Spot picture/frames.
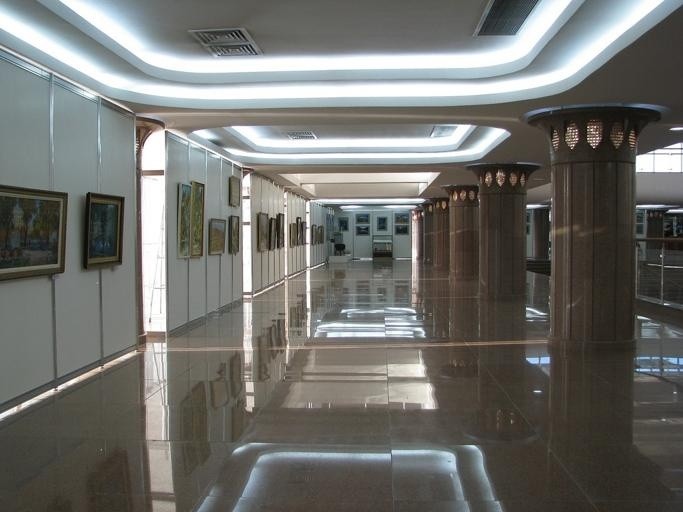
[177,176,410,259]
[84,191,125,270]
[46,269,410,512]
[0,184,68,281]
